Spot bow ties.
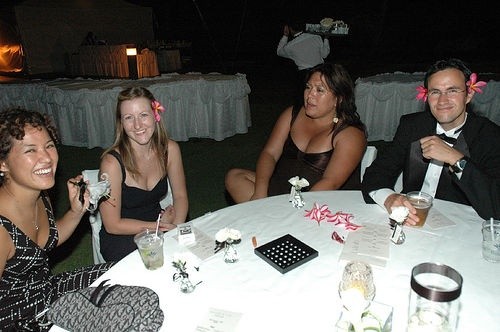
[435,133,457,145]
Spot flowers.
[215,227,241,244]
[304,202,366,230]
[386,205,409,224]
[416,85,429,103]
[75,173,115,211]
[465,73,487,94]
[151,100,166,121]
[288,176,310,190]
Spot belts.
[301,64,322,72]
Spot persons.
[277,15,330,93]
[225,63,367,204]
[361,56,500,230]
[0,108,99,332]
[98,86,189,262]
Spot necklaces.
[1,190,41,231]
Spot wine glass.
[339,262,376,332]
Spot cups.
[404,191,433,228]
[133,229,164,271]
[408,262,463,332]
[481,220,500,264]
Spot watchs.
[451,155,470,173]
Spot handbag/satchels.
[47,279,164,332]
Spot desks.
[354,71,500,142]
[48,190,500,332]
[0,72,252,150]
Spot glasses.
[425,89,466,98]
